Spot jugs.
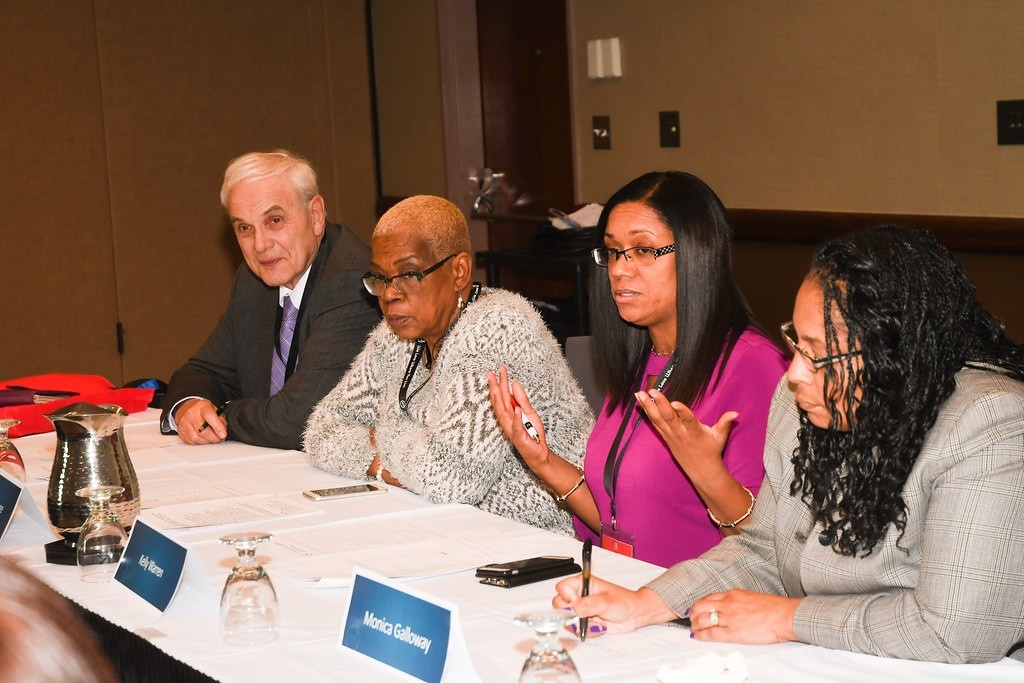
[43,401,142,549]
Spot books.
[0,389,80,407]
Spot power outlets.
[658,110,681,148]
[996,99,1024,146]
[591,114,612,151]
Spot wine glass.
[512,608,580,683]
[75,484,127,583]
[0,418,26,489]
[219,531,281,643]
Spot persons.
[486,172,793,570]
[159,148,383,451]
[552,227,1023,664]
[302,195,596,542]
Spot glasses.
[361,252,458,298]
[777,320,864,375]
[592,242,676,267]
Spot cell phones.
[303,484,388,500]
[477,557,574,576]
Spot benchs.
[724,208,1024,364]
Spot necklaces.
[651,345,675,355]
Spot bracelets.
[707,486,756,527]
[552,466,585,503]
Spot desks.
[0,391,1024,683]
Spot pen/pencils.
[581,537,593,643]
[510,394,540,443]
[198,400,232,432]
[6,386,25,389]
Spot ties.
[269,296,299,396]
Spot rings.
[711,611,719,626]
